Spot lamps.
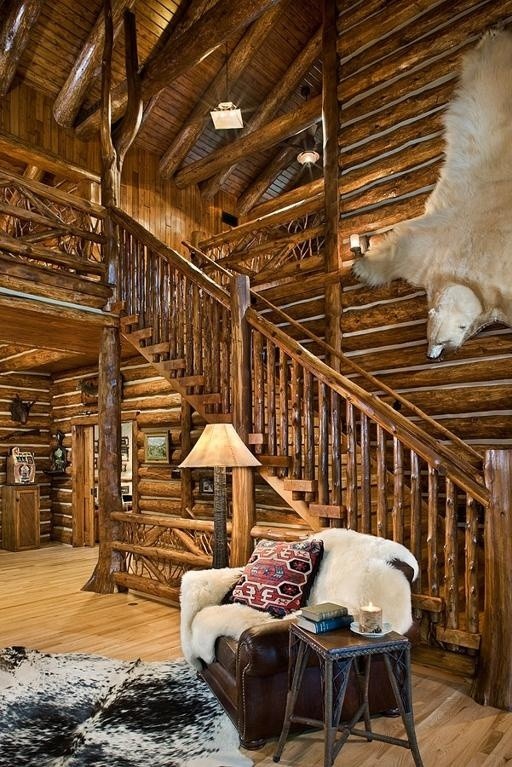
[349,232,365,259]
[176,422,264,571]
[296,147,321,169]
[208,39,246,132]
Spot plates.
[350,621,393,639]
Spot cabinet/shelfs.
[0,483,41,553]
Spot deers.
[9,394,39,425]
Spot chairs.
[179,527,419,751]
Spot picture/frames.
[140,427,171,465]
[198,477,215,497]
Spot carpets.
[1,647,256,767]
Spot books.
[300,601,348,620]
[297,614,354,634]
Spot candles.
[358,600,383,634]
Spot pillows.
[230,538,324,619]
